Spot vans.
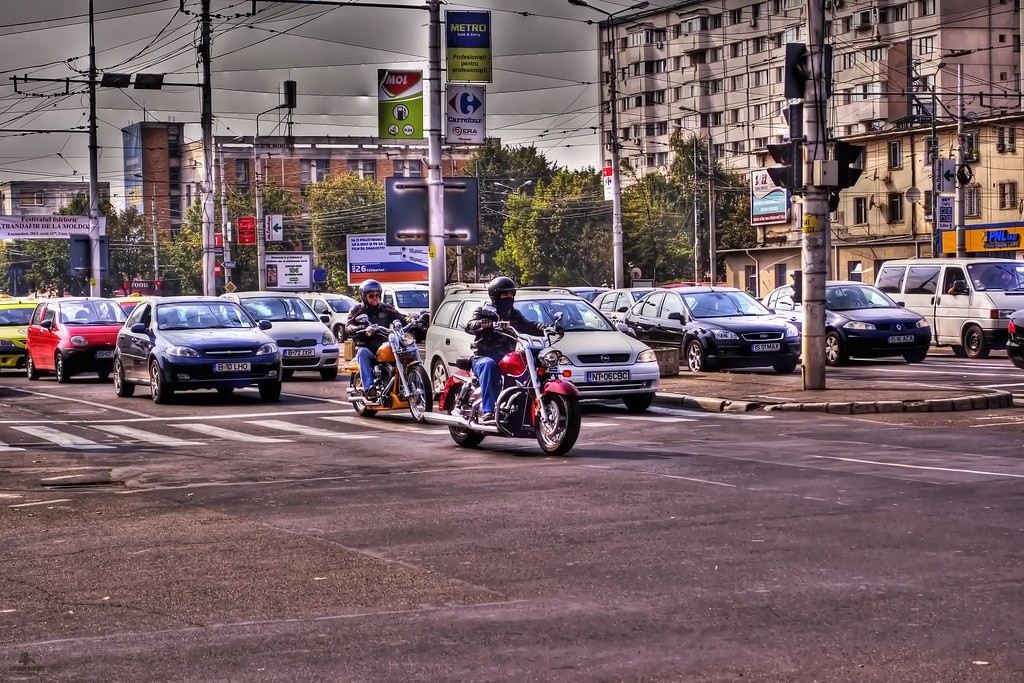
[871,256,1024,360]
[289,290,364,344]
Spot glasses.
[368,293,379,299]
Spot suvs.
[440,282,491,297]
[422,286,662,415]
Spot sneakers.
[477,412,496,424]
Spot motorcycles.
[339,309,434,424]
[421,310,581,458]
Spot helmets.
[488,277,518,309]
[358,279,382,304]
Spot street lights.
[253,102,293,294]
[928,63,949,257]
[678,106,720,287]
[566,0,653,288]
[670,121,701,287]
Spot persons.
[948,269,983,295]
[345,279,417,398]
[465,277,555,421]
[160,308,188,329]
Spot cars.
[111,295,284,402]
[760,280,932,366]
[217,290,339,381]
[580,288,680,332]
[0,294,42,370]
[1005,307,1024,372]
[109,291,159,321]
[376,281,431,319]
[549,285,615,305]
[621,285,801,374]
[25,295,129,383]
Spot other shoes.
[364,387,378,400]
